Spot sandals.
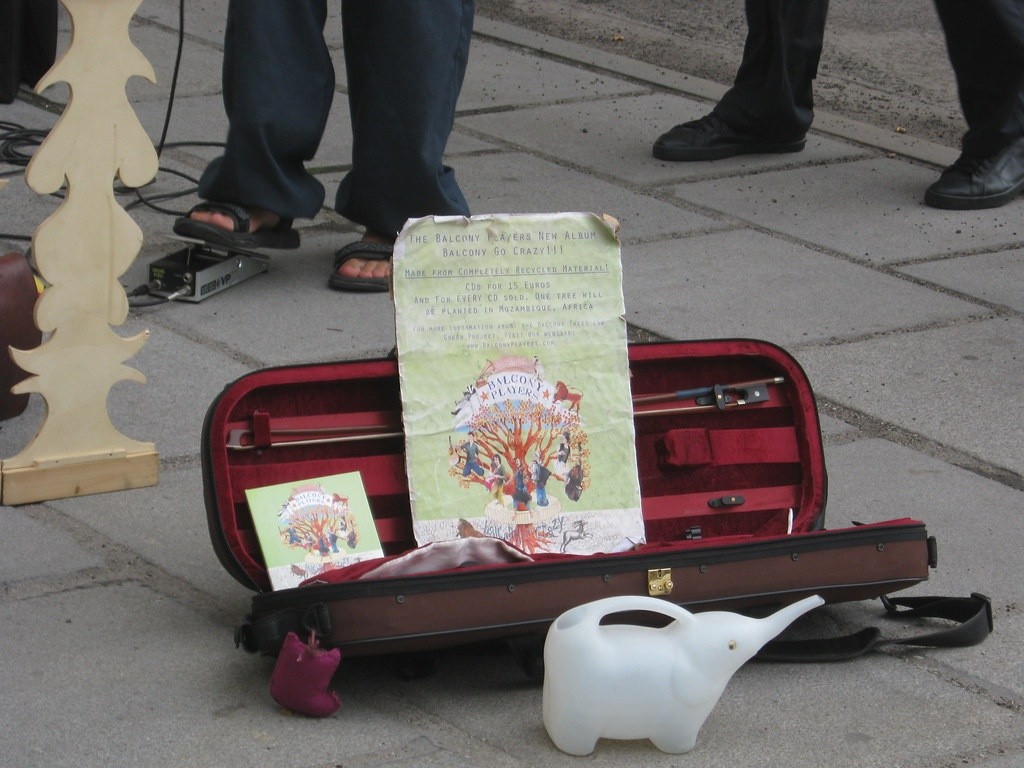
[330,241,396,293]
[172,199,303,254]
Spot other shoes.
[922,137,1024,211]
[652,120,808,163]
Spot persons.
[172,0,471,291]
[653,0,1024,209]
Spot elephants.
[541,593,827,758]
